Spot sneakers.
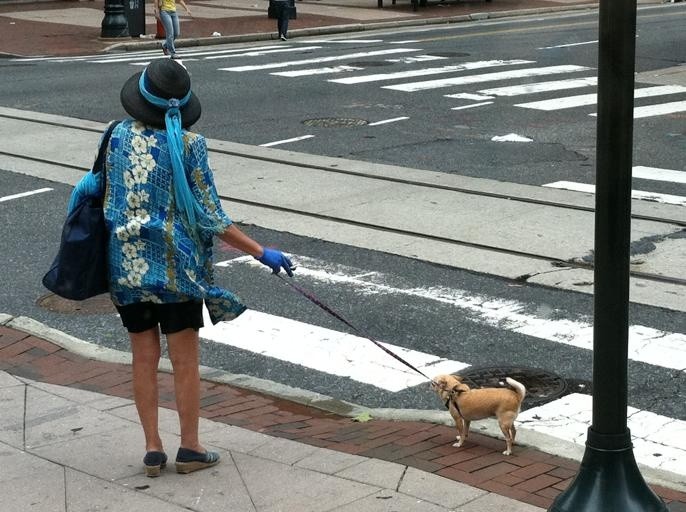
[281,33,287,41]
[162,47,180,58]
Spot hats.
[121,58,201,130]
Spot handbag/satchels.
[43,120,123,300]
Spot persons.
[270,1,292,41]
[91,58,298,479]
[153,1,194,59]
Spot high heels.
[175,448,221,474]
[143,451,168,477]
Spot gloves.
[254,247,296,276]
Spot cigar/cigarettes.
[289,264,298,269]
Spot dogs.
[428,371,527,457]
